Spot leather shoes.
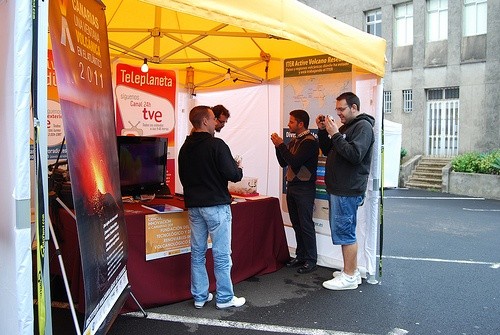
[286,258,305,267]
[298,263,317,274]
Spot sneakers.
[332,270,362,285]
[215,296,246,309]
[195,292,214,308]
[322,275,359,290]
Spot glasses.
[336,106,350,111]
[208,116,217,120]
[218,118,228,124]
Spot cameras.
[319,116,325,122]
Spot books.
[142,204,183,213]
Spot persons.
[271,109,319,274]
[190,104,230,133]
[315,92,375,290]
[178,105,245,309]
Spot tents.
[0,0,386,335]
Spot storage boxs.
[228,178,257,194]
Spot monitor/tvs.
[117,136,168,200]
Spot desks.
[48,190,290,313]
